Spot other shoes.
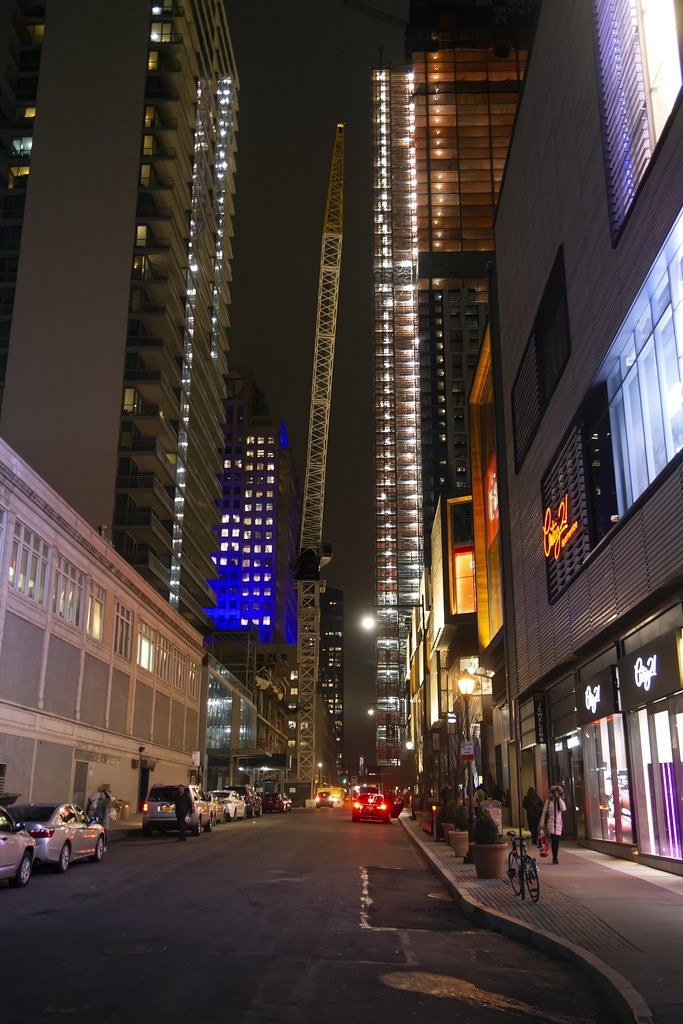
[553,858,559,864]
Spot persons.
[538,785,567,864]
[477,784,488,807]
[169,785,192,841]
[523,787,544,845]
[93,790,113,852]
[496,786,507,805]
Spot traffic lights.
[343,779,347,783]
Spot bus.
[304,798,344,809]
[316,786,348,797]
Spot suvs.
[224,786,262,818]
[142,783,216,838]
[213,789,247,822]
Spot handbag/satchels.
[536,808,548,824]
[538,833,549,857]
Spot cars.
[0,806,37,888]
[204,791,226,826]
[262,791,293,813]
[7,801,107,873]
[352,792,405,825]
[315,792,335,808]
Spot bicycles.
[505,830,540,902]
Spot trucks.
[252,780,312,807]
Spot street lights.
[458,668,480,865]
[406,741,417,819]
[318,760,322,786]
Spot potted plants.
[437,782,509,879]
